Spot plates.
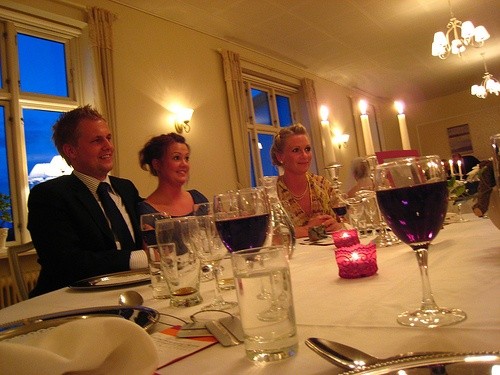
[337,349,500,375]
[0,304,161,346]
[69,270,160,289]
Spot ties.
[97,182,135,251]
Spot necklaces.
[284,176,309,200]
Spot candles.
[395,99,411,150]
[457,160,461,173]
[359,99,375,155]
[319,105,336,165]
[449,159,454,173]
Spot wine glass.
[323,165,404,248]
[211,186,273,277]
[179,182,294,314]
[373,153,467,329]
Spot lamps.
[471,52,500,98]
[431,0,490,60]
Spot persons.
[269,124,346,241]
[139,133,215,257]
[440,154,479,181]
[26,106,143,298]
[348,159,377,198]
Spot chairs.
[8,240,35,302]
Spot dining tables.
[0,213,500,375]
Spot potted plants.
[0,191,16,249]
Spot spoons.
[304,334,459,369]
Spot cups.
[152,217,205,307]
[139,213,178,299]
[489,132,500,186]
[230,246,300,363]
